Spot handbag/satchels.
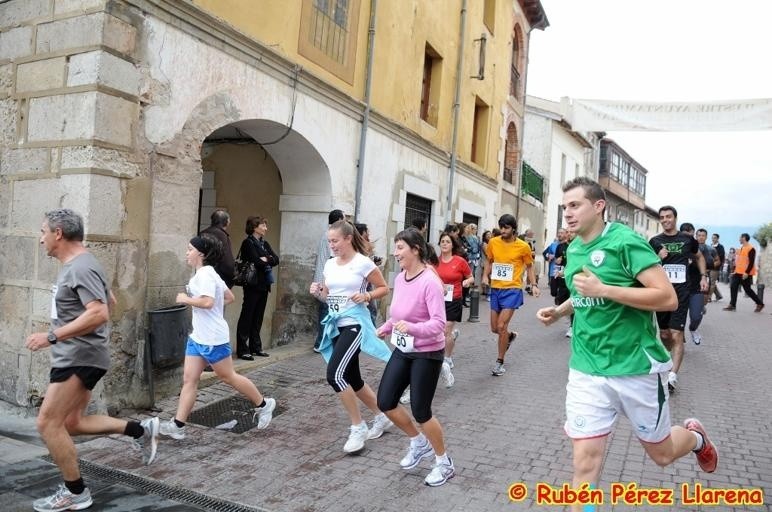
[234,261,258,286]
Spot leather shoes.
[754,304,764,311]
[238,351,269,360]
[724,304,735,311]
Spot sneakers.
[400,390,410,404]
[252,398,276,429]
[691,330,701,345]
[424,458,455,487]
[134,417,185,465]
[684,418,718,473]
[441,357,454,388]
[492,364,505,376]
[344,413,394,452]
[400,439,434,470]
[668,372,677,392]
[507,332,516,350]
[33,482,93,512]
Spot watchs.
[47,331,57,345]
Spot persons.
[309,218,394,454]
[375,229,455,487]
[24,208,159,512]
[158,234,277,442]
[235,213,280,361]
[535,175,718,512]
[199,210,236,291]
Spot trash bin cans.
[148,305,192,368]
